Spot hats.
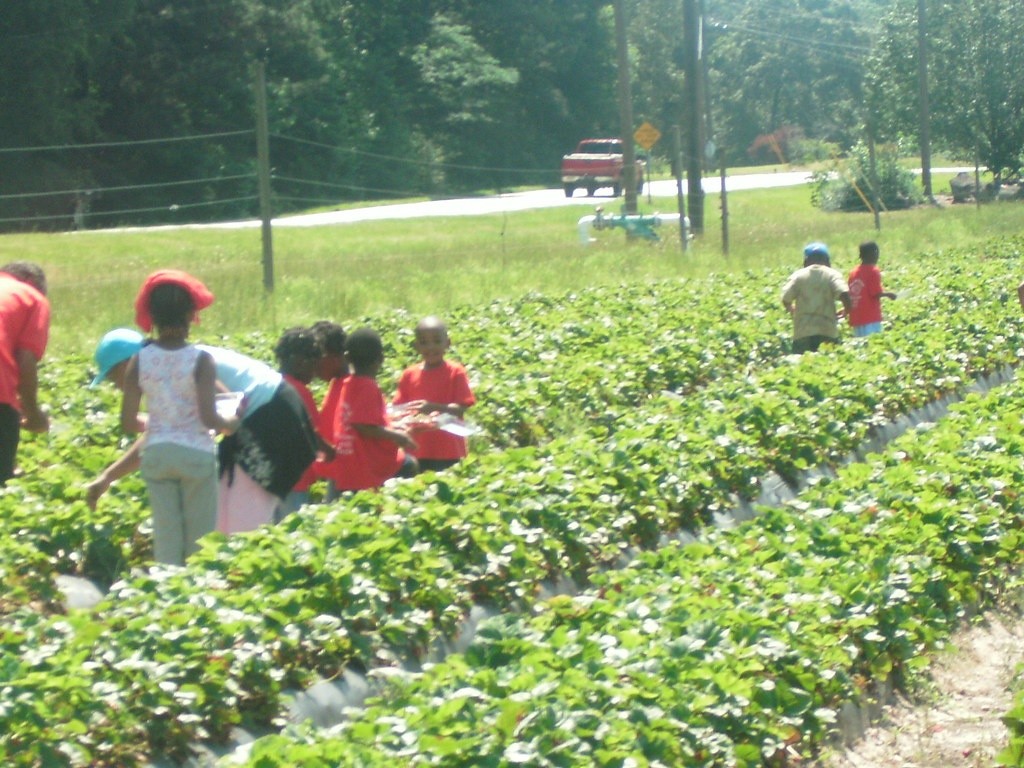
[90,327,145,393]
[804,244,830,257]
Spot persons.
[86,328,318,537]
[781,243,852,354]
[119,269,239,565]
[391,316,474,474]
[848,241,897,337]
[272,321,418,526]
[0,261,51,486]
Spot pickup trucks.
[561,138,647,197]
